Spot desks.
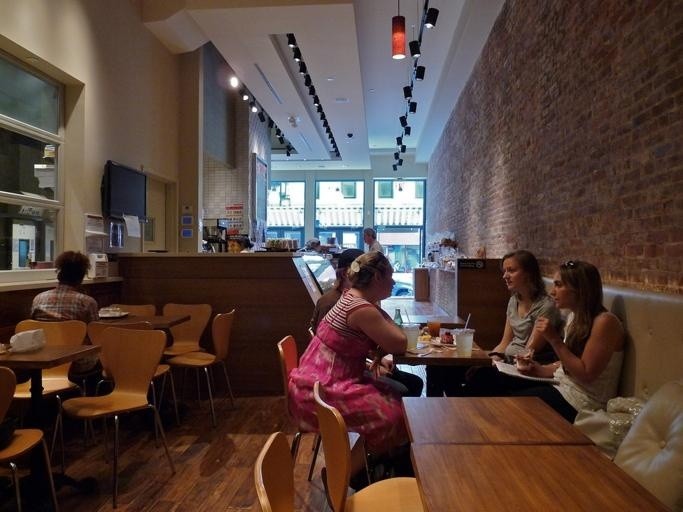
[1,314,194,402]
[401,396,595,444]
[410,442,667,510]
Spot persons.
[237,236,253,254]
[427,251,562,397]
[312,249,423,397]
[493,261,626,423]
[289,251,410,511]
[363,228,384,256]
[31,251,104,377]
[301,237,321,253]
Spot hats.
[338,247,365,269]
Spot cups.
[264,237,298,249]
[449,328,475,358]
[400,323,420,352]
[426,318,440,337]
[514,348,534,371]
[327,237,336,244]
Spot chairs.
[51,325,177,511]
[12,318,87,405]
[314,379,422,512]
[111,303,156,318]
[256,431,293,510]
[168,307,239,430]
[1,368,59,511]
[158,303,213,409]
[275,336,373,486]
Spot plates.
[430,339,456,347]
[259,247,300,253]
[98,311,131,319]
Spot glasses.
[565,260,580,286]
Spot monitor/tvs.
[100,160,147,224]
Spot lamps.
[228,75,291,156]
[285,37,338,160]
[392,0,439,171]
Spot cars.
[389,270,413,297]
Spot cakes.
[441,332,454,344]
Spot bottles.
[391,308,403,327]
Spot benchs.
[537,275,682,508]
